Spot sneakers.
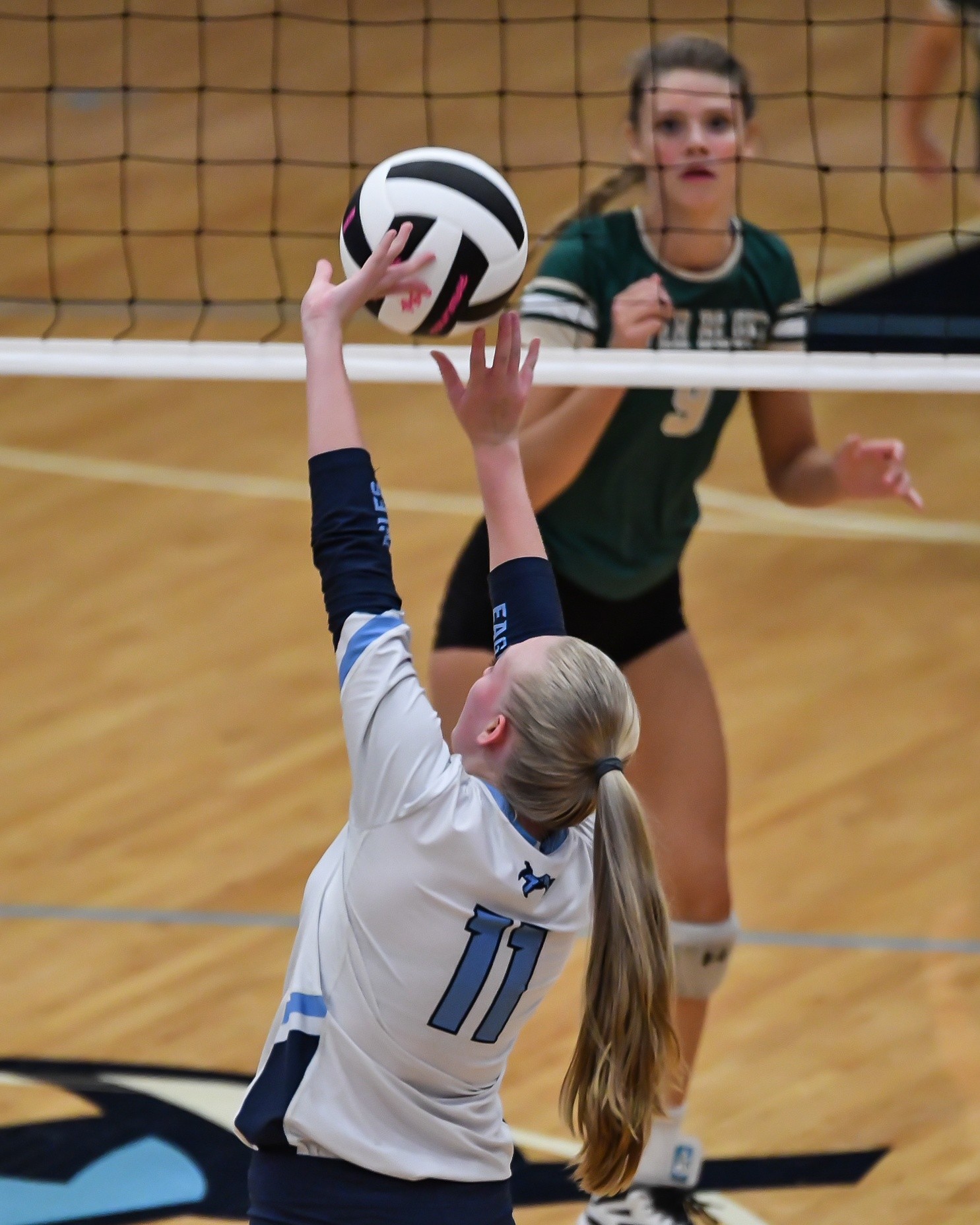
[576,1184,723,1225]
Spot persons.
[436,0,979,1225]
[232,221,680,1225]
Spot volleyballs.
[339,147,528,338]
